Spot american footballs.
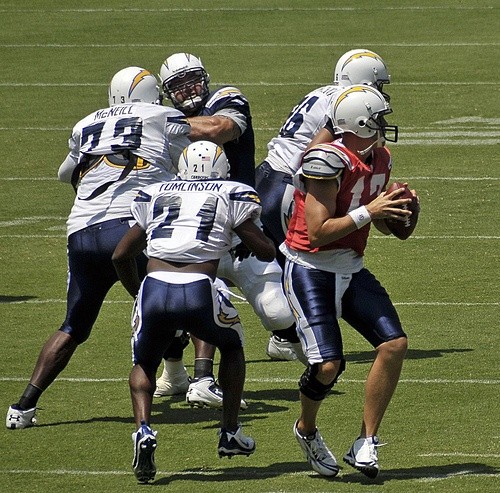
[384,182,418,241]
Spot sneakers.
[132,420,157,482]
[218,427,256,459]
[6,404,37,429]
[266,336,307,362]
[186,377,249,410]
[153,368,192,398]
[293,418,339,478]
[343,435,380,479]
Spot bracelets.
[349,205,371,229]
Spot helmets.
[177,140,231,180]
[331,85,393,138]
[333,48,391,84]
[157,52,210,89]
[108,66,163,105]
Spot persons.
[279,85,423,477]
[6,48,391,429]
[112,142,276,482]
